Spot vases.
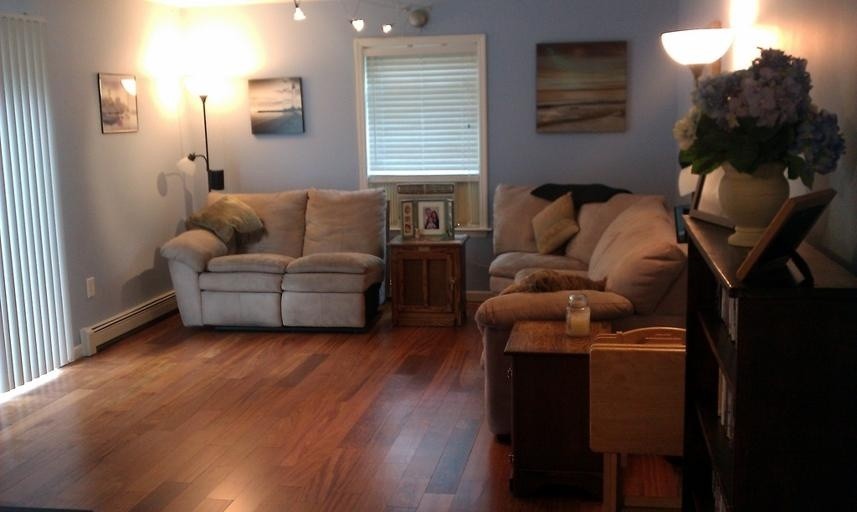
[711,155,789,248]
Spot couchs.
[477,180,700,443]
[161,187,391,330]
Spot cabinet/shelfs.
[677,210,857,511]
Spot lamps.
[174,82,227,195]
[658,24,729,84]
[290,0,394,36]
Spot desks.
[386,230,467,324]
[504,320,613,500]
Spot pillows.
[497,267,606,298]
[529,190,582,253]
[186,198,267,241]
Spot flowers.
[669,47,844,185]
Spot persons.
[425,207,439,228]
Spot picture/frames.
[97,71,139,135]
[738,188,840,282]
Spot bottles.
[564,293,592,338]
